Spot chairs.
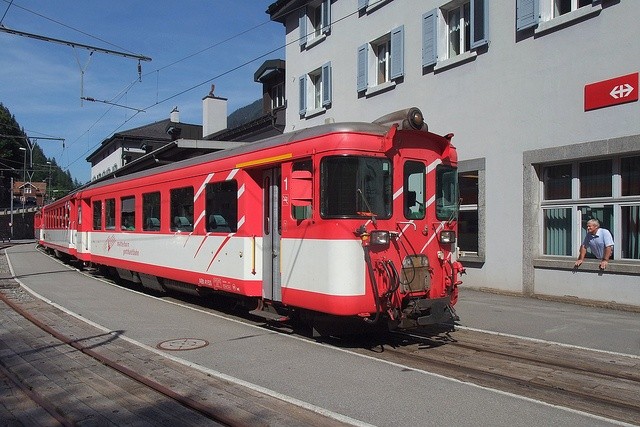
[108,217,115,226]
[209,215,232,232]
[125,216,134,227]
[97,219,101,228]
[174,216,193,231]
[146,217,160,230]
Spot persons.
[574,219,614,270]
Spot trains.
[34,106,465,347]
